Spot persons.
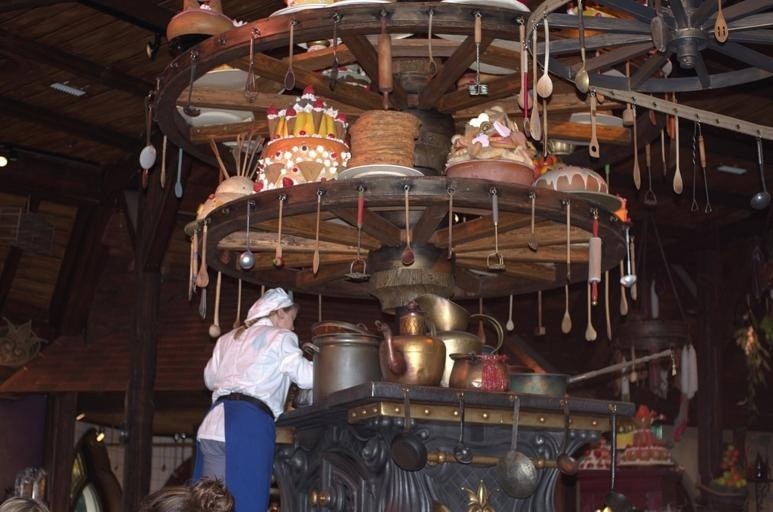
[0,495,49,512]
[191,286,314,511]
[139,478,233,512]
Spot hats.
[244,287,294,322]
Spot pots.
[449,352,510,391]
[390,390,427,471]
[301,321,380,404]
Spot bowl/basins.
[444,159,536,187]
[508,372,570,395]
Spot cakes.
[531,154,610,193]
[196,176,256,220]
[444,105,537,170]
[577,439,613,471]
[613,195,631,222]
[617,429,674,467]
[253,84,350,192]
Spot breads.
[347,109,423,170]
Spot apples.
[716,444,747,488]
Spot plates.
[339,164,424,178]
[561,190,622,214]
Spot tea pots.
[414,293,504,385]
[373,302,447,386]
